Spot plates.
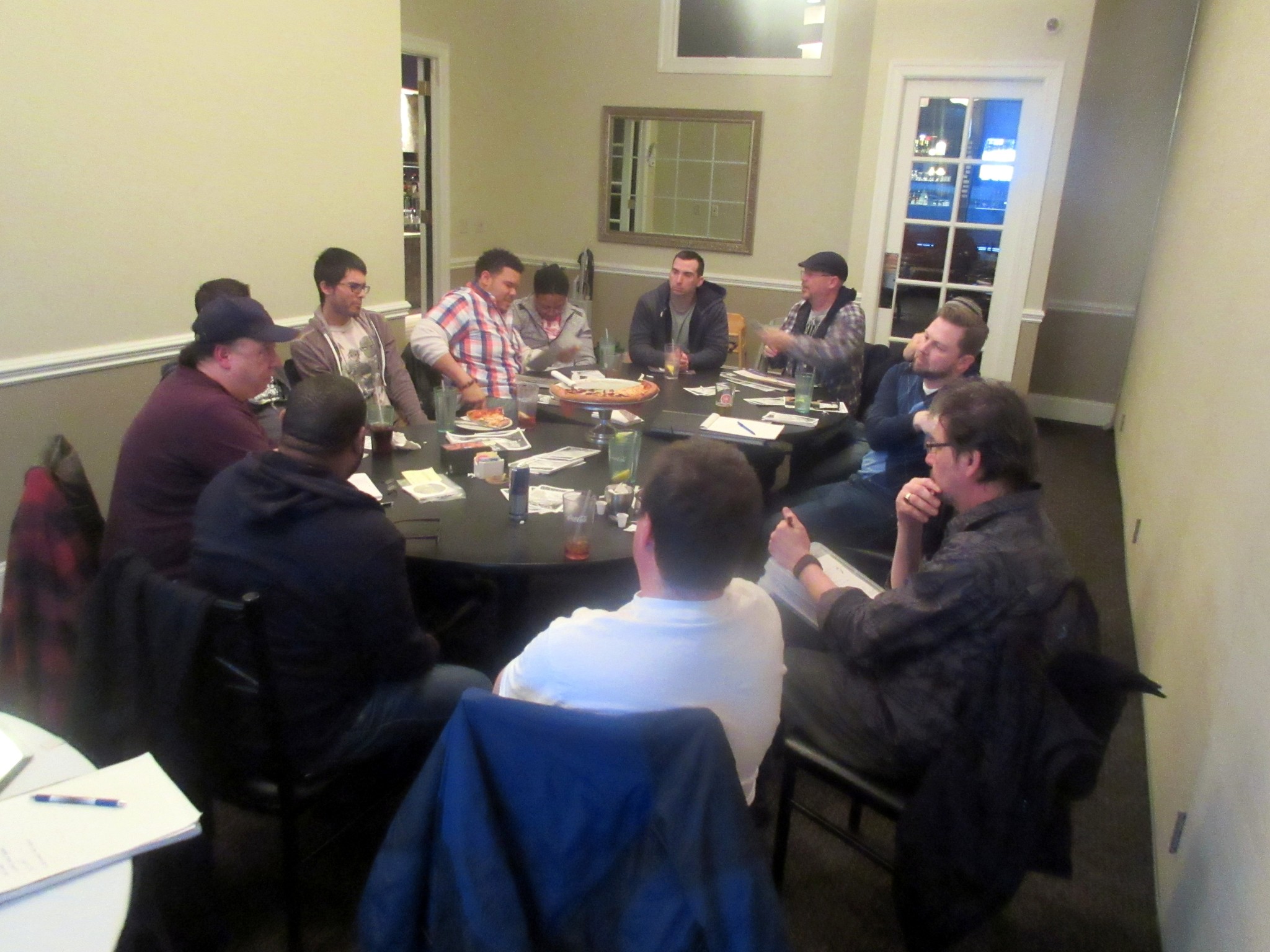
[454,414,513,431]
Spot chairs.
[1,313,1127,952]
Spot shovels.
[551,370,583,391]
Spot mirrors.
[598,106,764,256]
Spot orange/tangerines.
[720,394,733,404]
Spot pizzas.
[466,406,510,429]
[550,380,658,402]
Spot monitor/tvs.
[658,0,836,79]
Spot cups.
[605,353,622,379]
[367,405,395,458]
[433,385,458,433]
[715,382,736,407]
[617,513,628,528]
[599,337,615,369]
[605,484,634,516]
[609,429,642,484]
[596,501,607,515]
[558,335,582,363]
[664,343,683,380]
[795,372,816,413]
[517,383,539,430]
[563,490,596,560]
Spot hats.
[798,251,848,281]
[192,295,299,344]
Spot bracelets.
[457,378,479,390]
[792,553,823,580]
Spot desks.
[516,363,853,503]
[347,423,675,668]
[0,711,133,952]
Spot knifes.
[455,422,499,430]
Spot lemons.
[795,399,805,406]
[666,364,674,375]
[615,431,633,443]
[611,469,630,482]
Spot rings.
[904,492,911,502]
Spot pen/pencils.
[380,501,394,506]
[29,794,127,807]
[788,516,794,528]
[737,421,757,435]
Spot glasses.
[325,280,370,293]
[924,438,954,454]
[801,271,836,280]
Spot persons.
[513,263,596,368]
[754,252,1074,790]
[187,377,491,804]
[626,250,729,371]
[98,277,302,585]
[491,442,788,806]
[408,247,529,407]
[291,249,431,427]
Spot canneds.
[508,462,530,519]
